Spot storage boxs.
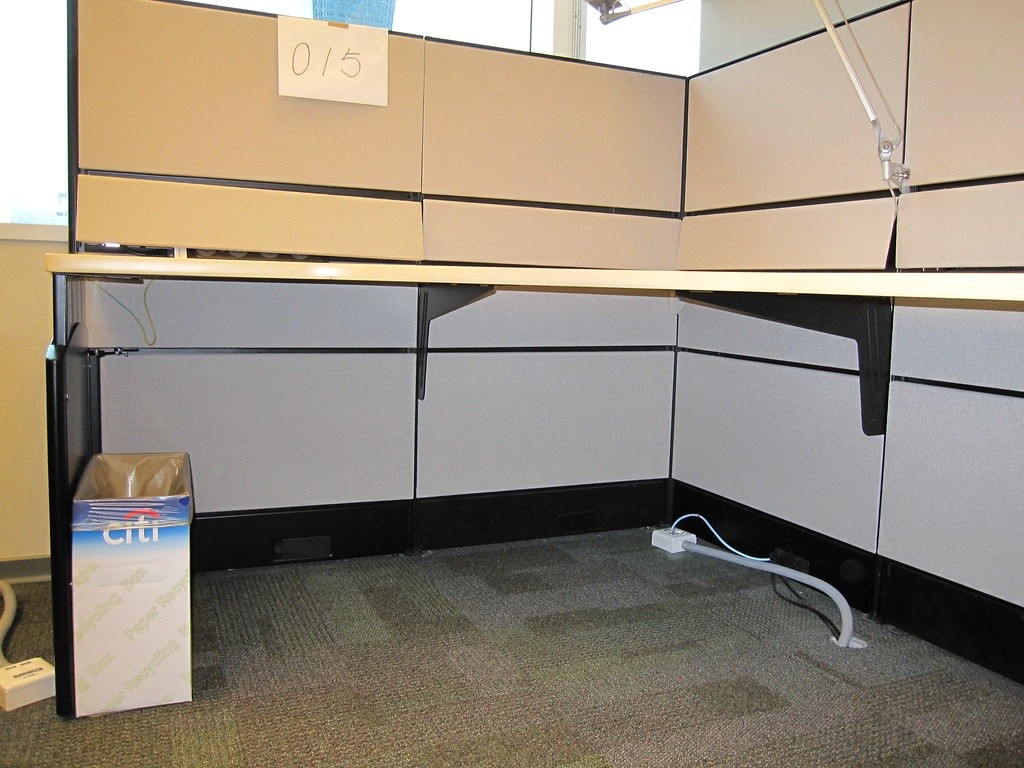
[71,453,193,719]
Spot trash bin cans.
[72,451,196,717]
